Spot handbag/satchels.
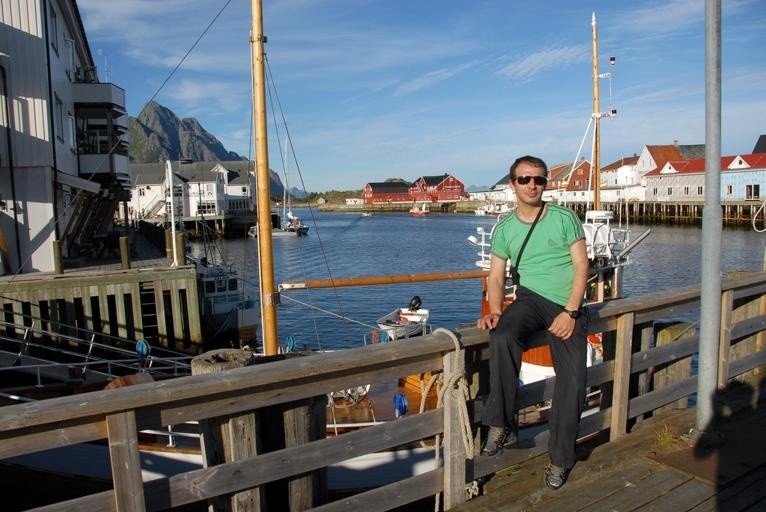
[507,264,521,286]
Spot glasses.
[510,175,547,187]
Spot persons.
[478,155,590,491]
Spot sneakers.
[542,460,569,491]
[477,423,519,460]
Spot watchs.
[563,309,579,318]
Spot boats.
[473,203,514,215]
[376,295,431,341]
[362,212,372,216]
[187,182,261,341]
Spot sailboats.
[408,197,431,217]
[106,2,651,492]
[247,130,310,238]
